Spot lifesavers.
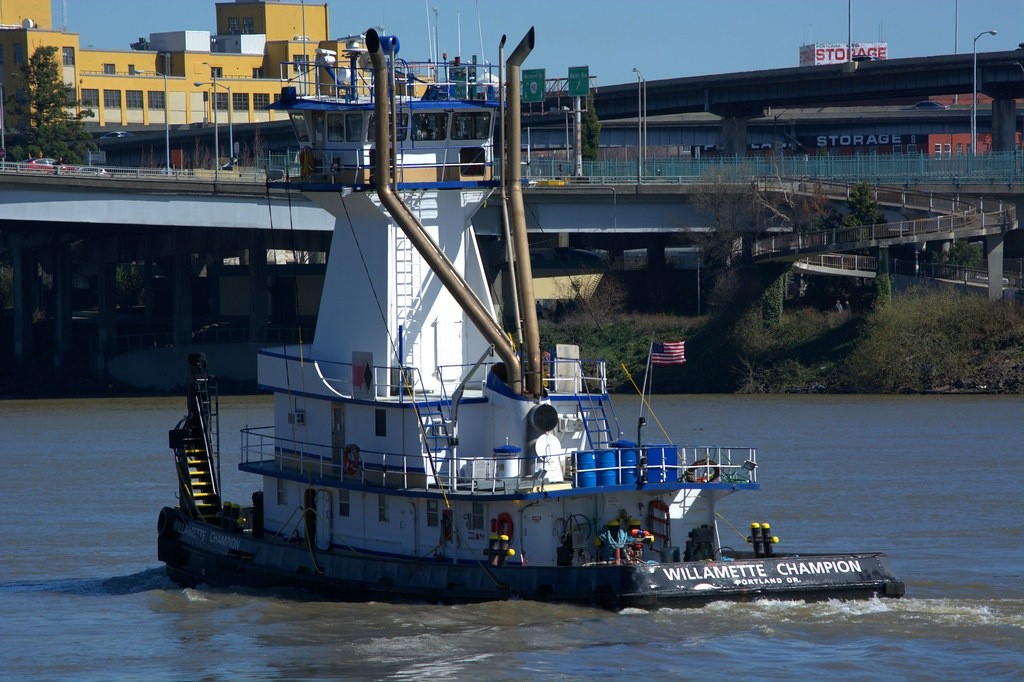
[685,458,720,483]
[156,506,175,537]
[299,146,315,177]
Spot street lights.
[634,68,646,177]
[192,81,235,162]
[133,68,172,176]
[970,30,999,154]
[202,62,220,180]
[632,66,643,182]
[560,104,572,160]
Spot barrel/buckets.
[594,448,617,486]
[660,546,681,563]
[616,449,637,484]
[571,451,597,488]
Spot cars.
[18,158,75,171]
[95,131,133,139]
[75,165,109,176]
[851,55,882,62]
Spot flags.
[652,341,686,364]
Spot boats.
[152,22,910,606]
